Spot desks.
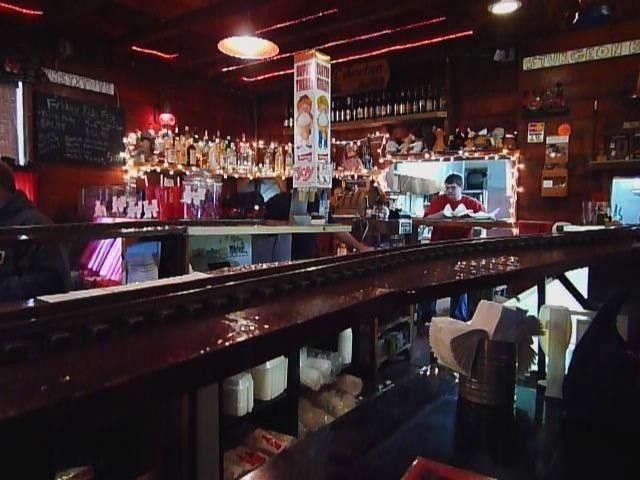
[240,364,639,480]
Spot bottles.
[331,81,447,123]
[124,121,295,177]
[283,104,294,127]
[88,184,146,218]
[522,81,568,111]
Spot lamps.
[487,0,523,16]
[216,0,279,60]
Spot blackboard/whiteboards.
[32,90,125,166]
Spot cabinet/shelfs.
[282,108,522,162]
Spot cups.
[338,328,353,364]
[300,358,332,391]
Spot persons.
[413,174,484,326]
[264,176,375,262]
[0,161,70,303]
